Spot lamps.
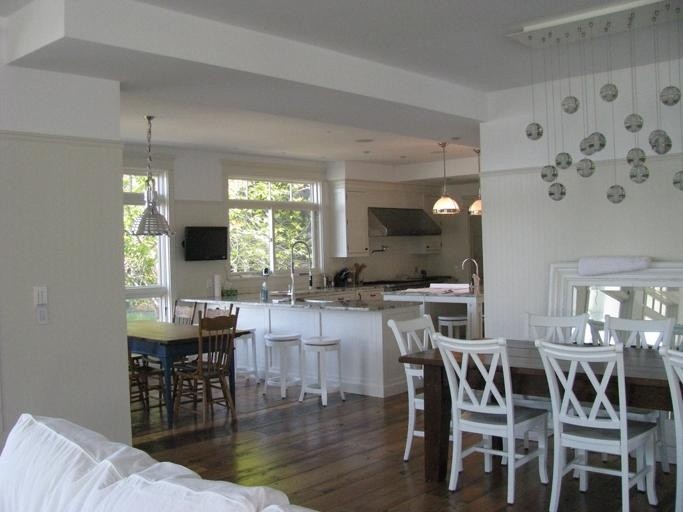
[468,148,483,216]
[431,143,460,215]
[132,115,174,237]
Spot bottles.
[321,274,334,288]
[407,269,427,280]
[260,282,267,300]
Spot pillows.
[0,413,290,512]
[259,503,318,512]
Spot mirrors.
[546,259,683,347]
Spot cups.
[213,275,221,297]
[351,271,362,286]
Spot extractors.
[367,206,442,238]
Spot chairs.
[127,300,242,429]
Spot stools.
[234,327,261,384]
[300,337,345,406]
[262,331,304,399]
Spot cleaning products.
[259,267,273,303]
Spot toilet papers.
[213,273,222,299]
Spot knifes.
[337,268,350,281]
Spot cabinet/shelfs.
[327,291,357,301]
[297,293,327,300]
[356,288,384,300]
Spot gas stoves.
[364,280,426,292]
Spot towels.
[578,256,652,275]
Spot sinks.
[296,296,334,307]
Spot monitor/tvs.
[184,226,228,260]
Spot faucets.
[461,256,480,298]
[290,240,314,306]
